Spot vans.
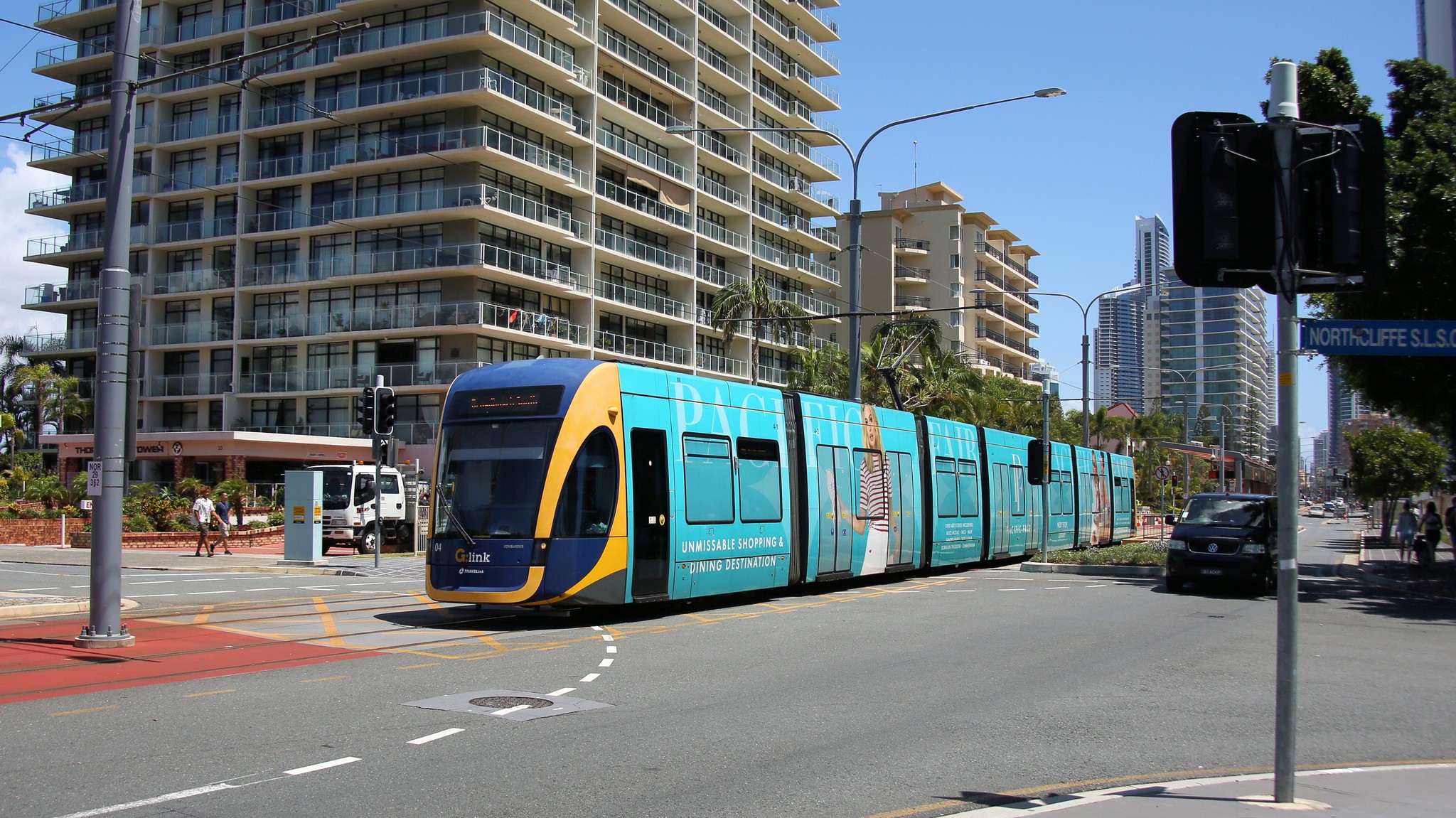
[1165,491,1280,596]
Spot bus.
[430,358,1134,606]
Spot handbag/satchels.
[190,516,199,526]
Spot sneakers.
[210,544,214,553]
[224,550,232,555]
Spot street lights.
[1107,361,1306,512]
[967,280,1183,449]
[1297,436,1345,500]
[666,86,1068,404]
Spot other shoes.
[195,552,204,556]
[208,553,214,557]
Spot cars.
[1323,497,1344,513]
[1306,505,1325,518]
[1299,494,1329,506]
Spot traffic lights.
[374,386,395,435]
[1171,488,1176,495]
[1164,480,1168,486]
[357,386,375,436]
[1171,475,1178,488]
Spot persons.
[1444,496,1456,560]
[1417,501,1443,575]
[1090,449,1109,545]
[209,491,232,555]
[1394,500,1417,563]
[834,404,900,576]
[193,486,223,557]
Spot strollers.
[1410,528,1445,565]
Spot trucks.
[308,460,441,555]
